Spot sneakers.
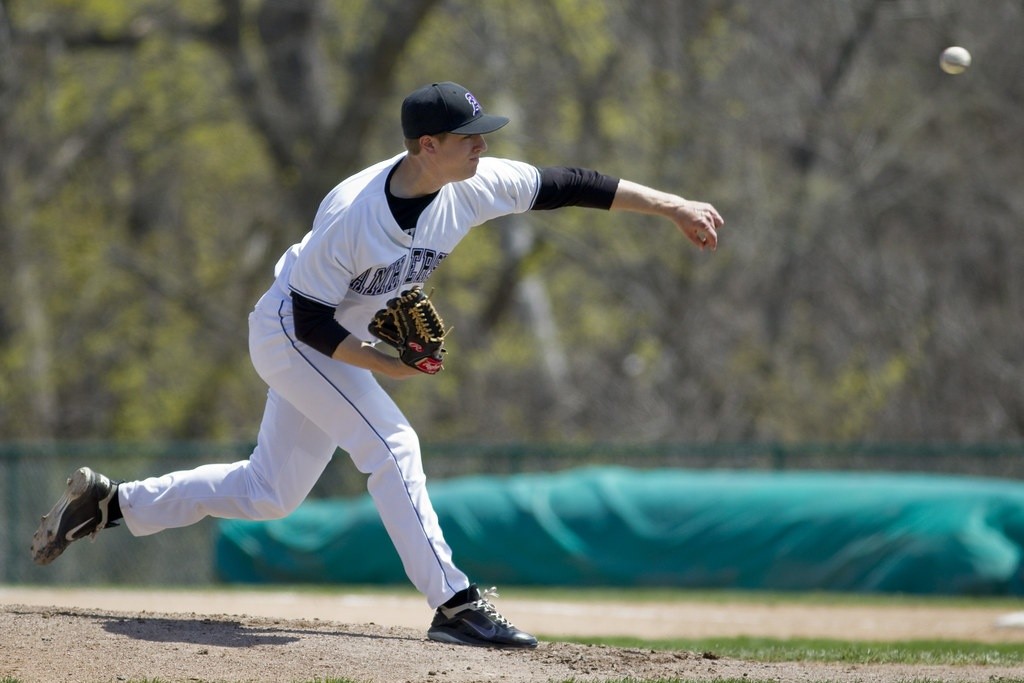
[30,466,125,565]
[427,583,538,650]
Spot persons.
[31,82,725,651]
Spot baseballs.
[940,45,972,75]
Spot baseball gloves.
[367,285,455,375]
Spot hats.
[401,81,511,139]
